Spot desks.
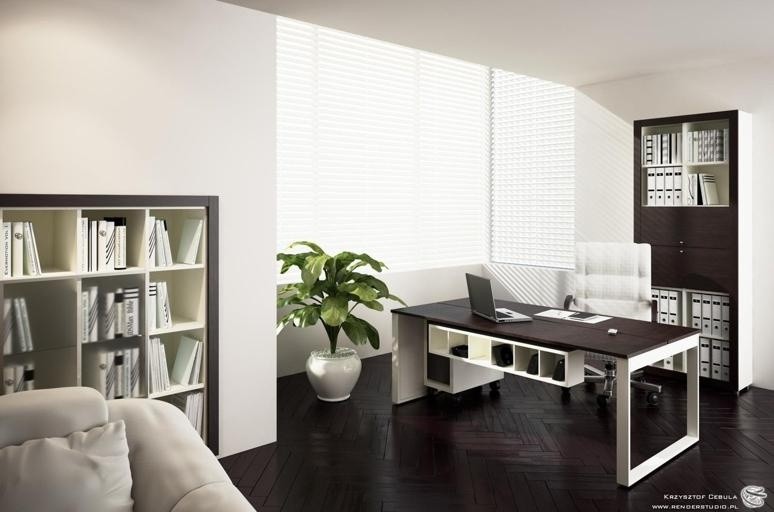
[390,295,701,488]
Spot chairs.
[1,385,257,512]
[560,240,663,420]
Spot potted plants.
[274,241,408,404]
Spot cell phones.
[608,328,618,336]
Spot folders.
[647,166,681,208]
[641,133,680,164]
[688,128,723,161]
[650,286,730,384]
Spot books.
[642,126,728,206]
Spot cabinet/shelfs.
[631,107,754,400]
[0,205,209,448]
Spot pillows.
[0,420,136,511]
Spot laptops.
[466,273,533,323]
